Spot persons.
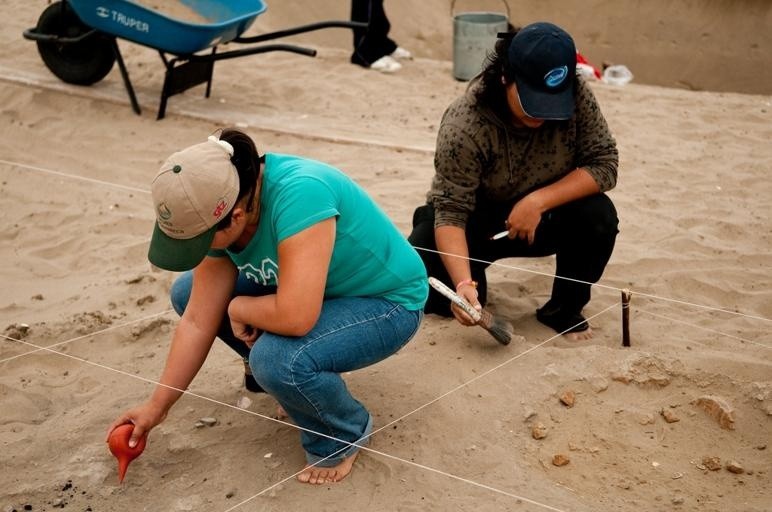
[408,22,620,343]
[105,128,430,485]
[350,0,413,74]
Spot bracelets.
[455,281,479,291]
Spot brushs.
[429,277,512,347]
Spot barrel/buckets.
[450,13,507,80]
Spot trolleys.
[23,1,370,121]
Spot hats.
[148,139,242,272]
[508,22,577,122]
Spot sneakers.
[370,55,402,75]
[391,46,414,63]
